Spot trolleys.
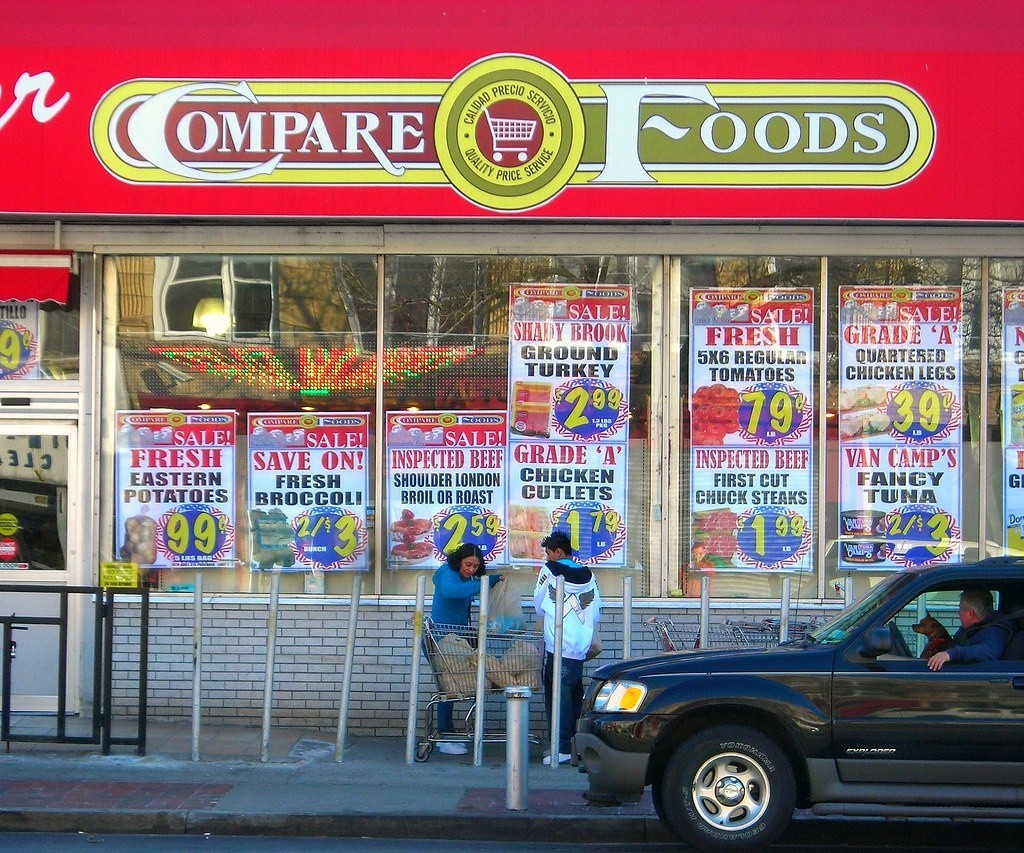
[643,580,929,656]
[407,613,545,764]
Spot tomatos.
[691,384,740,445]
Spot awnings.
[0,248,80,305]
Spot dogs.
[912,608,953,659]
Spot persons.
[421,544,507,754]
[926,588,1013,670]
[534,529,601,765]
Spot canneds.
[840,542,886,563]
[841,510,886,536]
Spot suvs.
[568,554,1024,853]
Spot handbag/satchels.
[466,627,478,651]
[487,577,526,638]
[582,627,602,662]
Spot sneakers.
[543,752,572,764]
[439,743,468,754]
[436,743,466,748]
